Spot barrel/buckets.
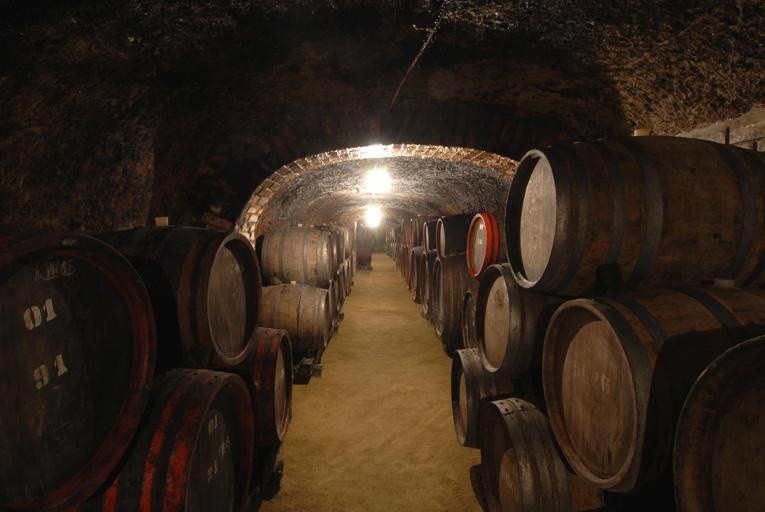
[386,136,765,511]
[0,225,354,512]
